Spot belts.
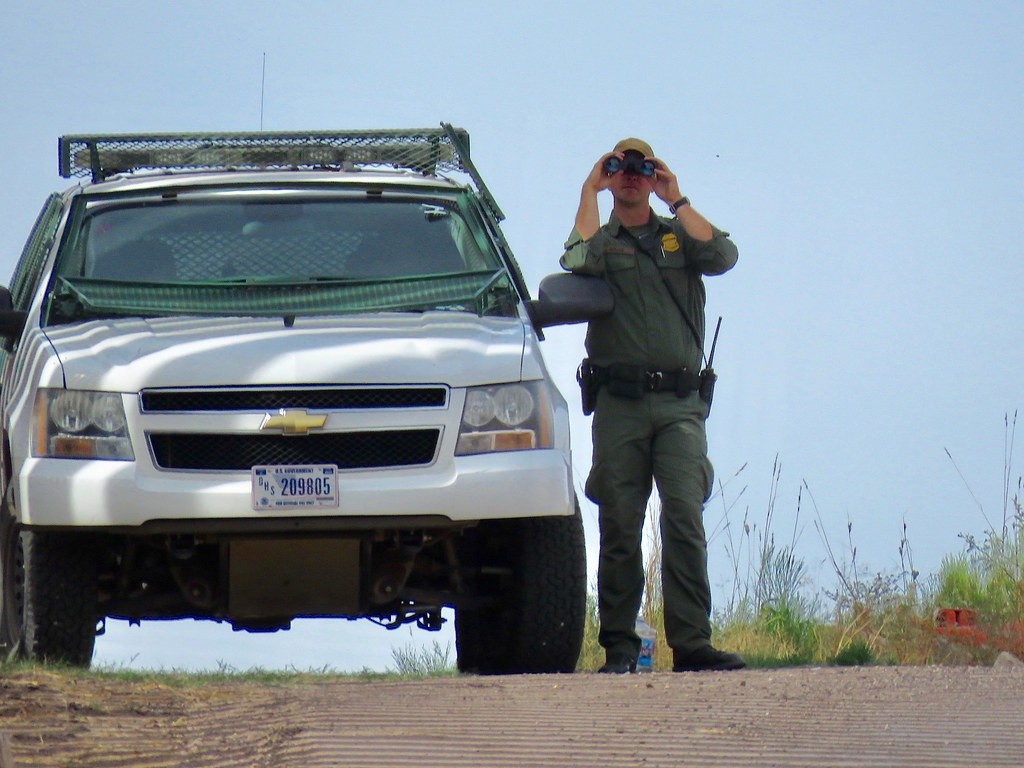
[647,371,678,392]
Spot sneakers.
[672,646,745,672]
[598,656,636,674]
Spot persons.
[557,137,747,673]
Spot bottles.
[634,613,657,672]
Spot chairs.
[345,228,466,277]
[89,240,178,281]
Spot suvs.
[0,123,588,677]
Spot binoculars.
[603,155,657,177]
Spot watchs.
[669,196,691,214]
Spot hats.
[613,138,655,157]
